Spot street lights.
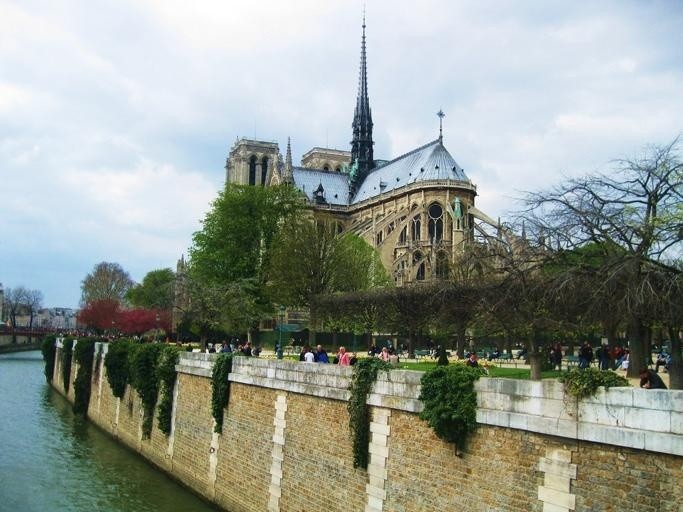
[275,305,287,359]
[156,312,161,343]
